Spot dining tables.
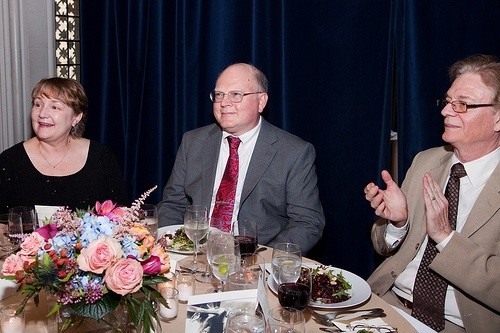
[1,223,415,333]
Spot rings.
[430,196,436,201]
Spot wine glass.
[184,203,312,333]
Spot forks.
[308,306,384,322]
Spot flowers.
[0,185,176,333]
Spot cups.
[138,205,159,240]
[224,308,266,333]
[158,287,179,321]
[155,271,175,294]
[0,205,35,261]
[268,306,306,333]
[0,303,26,333]
[176,273,195,304]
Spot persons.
[0,76,129,225]
[363,53,500,333]
[153,63,326,257]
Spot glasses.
[436,98,496,114]
[209,90,264,104]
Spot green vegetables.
[311,264,352,297]
[172,226,207,251]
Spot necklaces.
[37,136,71,169]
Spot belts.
[393,290,413,310]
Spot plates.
[156,223,223,255]
[268,266,370,308]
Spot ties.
[210,136,242,234]
[411,163,468,332]
